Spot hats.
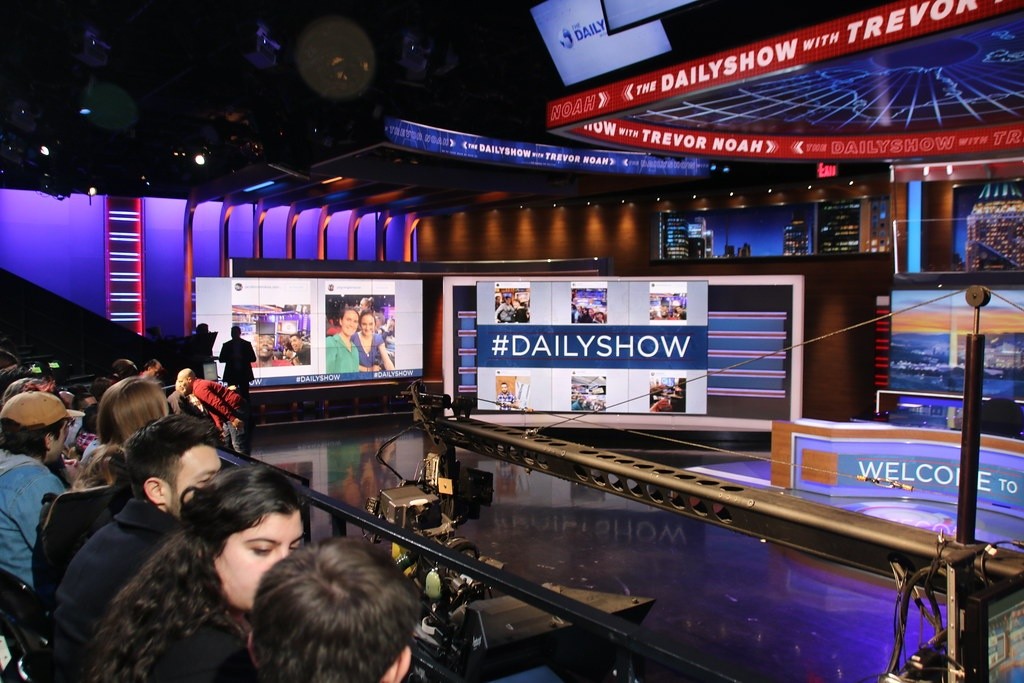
[0,391,85,434]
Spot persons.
[220,326,256,397]
[191,324,220,378]
[325,297,394,374]
[53,416,220,683]
[495,296,528,323]
[251,333,310,367]
[168,368,260,455]
[498,383,514,410]
[571,303,607,324]
[0,391,85,600]
[84,467,304,683]
[249,537,421,683]
[0,330,163,467]
[653,303,686,320]
[71,378,168,492]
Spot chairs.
[979,398,1023,435]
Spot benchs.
[247,380,399,424]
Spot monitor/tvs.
[530,0,712,86]
[963,571,1024,683]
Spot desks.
[771,419,1024,519]
